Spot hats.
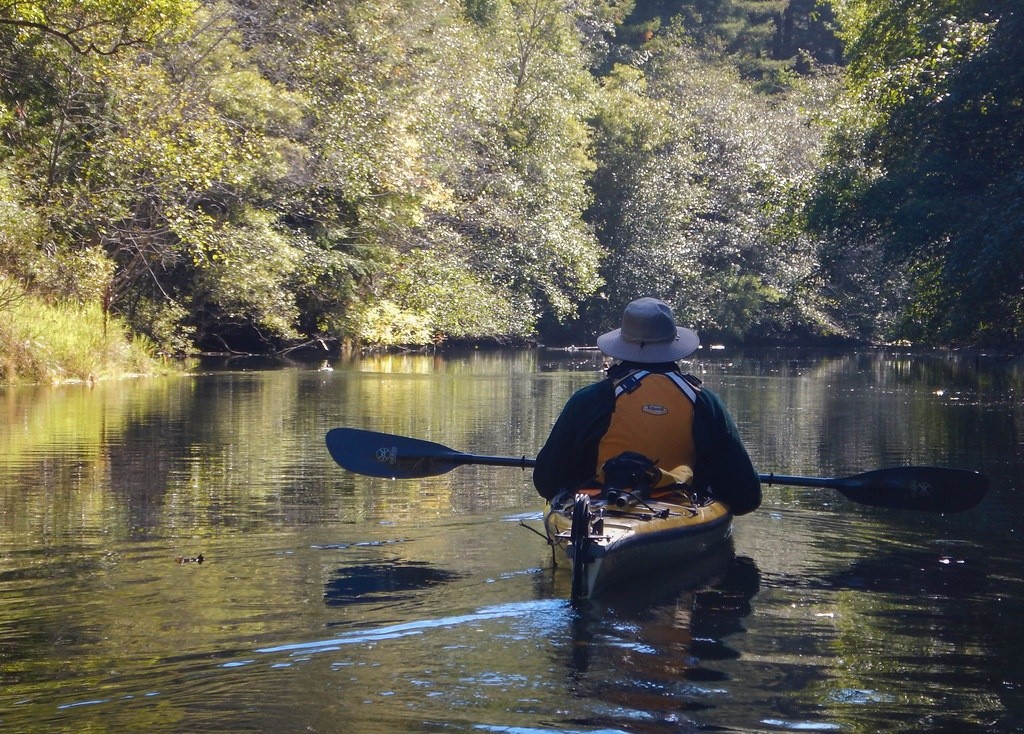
[596,296,700,364]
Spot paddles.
[323,423,995,520]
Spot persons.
[530,299,762,516]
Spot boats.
[541,451,734,600]
